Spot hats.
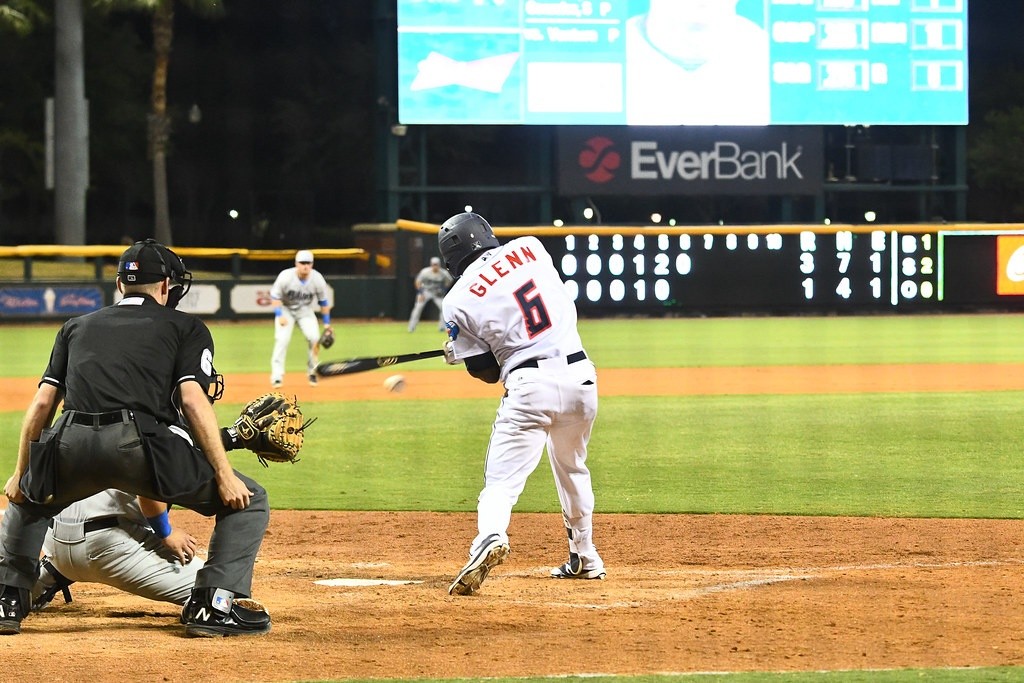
[295,250,315,262]
[430,257,440,265]
[118,239,175,285]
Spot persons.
[28,392,319,613]
[271,250,331,387]
[406,257,454,333]
[438,212,606,596]
[0,238,271,639]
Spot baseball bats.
[316,349,445,377]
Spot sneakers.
[179,587,272,636]
[447,533,511,596]
[0,584,25,633]
[551,554,608,581]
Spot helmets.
[437,213,499,280]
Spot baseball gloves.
[319,328,334,348]
[234,392,304,464]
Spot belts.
[511,351,587,371]
[49,518,118,534]
[67,409,133,426]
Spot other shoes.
[309,375,317,386]
[272,380,281,389]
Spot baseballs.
[384,375,405,393]
